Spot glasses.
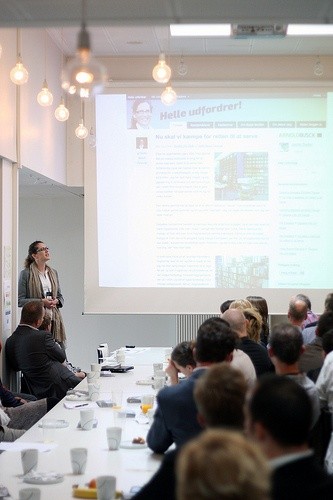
[33,246,48,254]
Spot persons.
[146,292,333,500]
[1,301,87,442]
[18,241,66,347]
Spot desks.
[0,347,176,500]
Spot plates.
[66,396,89,401]
[24,471,64,483]
[38,420,70,428]
[120,439,148,449]
[136,379,152,384]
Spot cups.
[71,447,87,475]
[19,488,41,500]
[115,350,125,365]
[141,393,154,413]
[111,390,123,409]
[85,364,101,401]
[95,476,116,500]
[42,418,56,444]
[80,407,95,431]
[107,426,121,451]
[153,349,172,393]
[21,449,38,474]
